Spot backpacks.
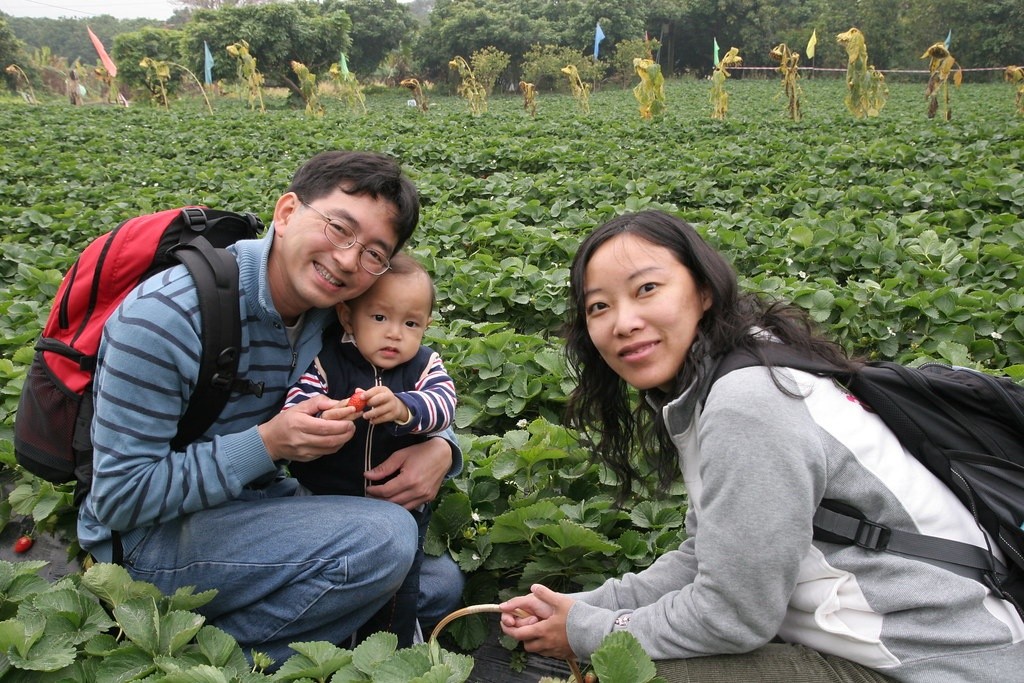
[698,319,1023,619]
[12,205,265,566]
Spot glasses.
[301,199,393,276]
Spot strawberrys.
[346,391,368,412]
[15,534,32,553]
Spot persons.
[72,148,462,678]
[282,254,457,651]
[501,212,1024,683]
